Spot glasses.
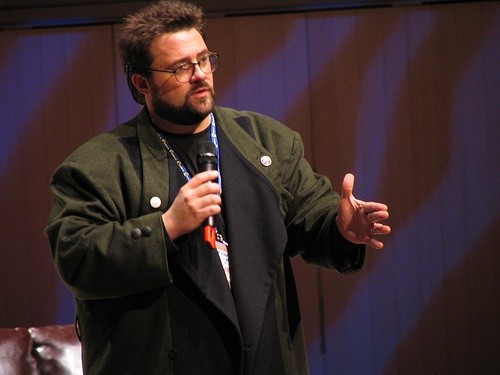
[138,50,219,84]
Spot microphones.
[196,139,219,249]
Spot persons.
[44,2,392,375]
